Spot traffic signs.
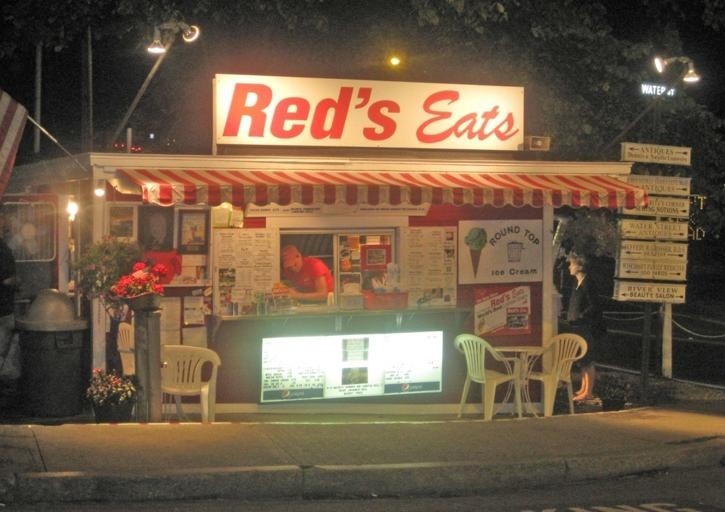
[617,239,689,263]
[615,260,686,279]
[627,175,692,195]
[620,141,692,167]
[617,217,688,241]
[613,279,686,304]
[620,197,690,218]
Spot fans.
[2,199,58,265]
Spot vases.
[92,403,133,423]
[116,291,162,310]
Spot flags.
[0,88,29,203]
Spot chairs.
[161,344,222,423]
[116,323,138,384]
[454,333,515,424]
[516,333,588,418]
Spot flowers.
[84,366,139,407]
[111,259,169,301]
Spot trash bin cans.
[14,290,94,424]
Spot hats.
[280,244,298,268]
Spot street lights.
[110,10,204,144]
[601,50,707,156]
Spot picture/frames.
[176,207,211,256]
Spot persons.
[584,237,616,335]
[554,237,576,311]
[272,244,334,303]
[0,236,23,424]
[144,211,173,251]
[567,245,602,401]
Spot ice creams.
[463,227,488,280]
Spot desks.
[489,346,545,418]
